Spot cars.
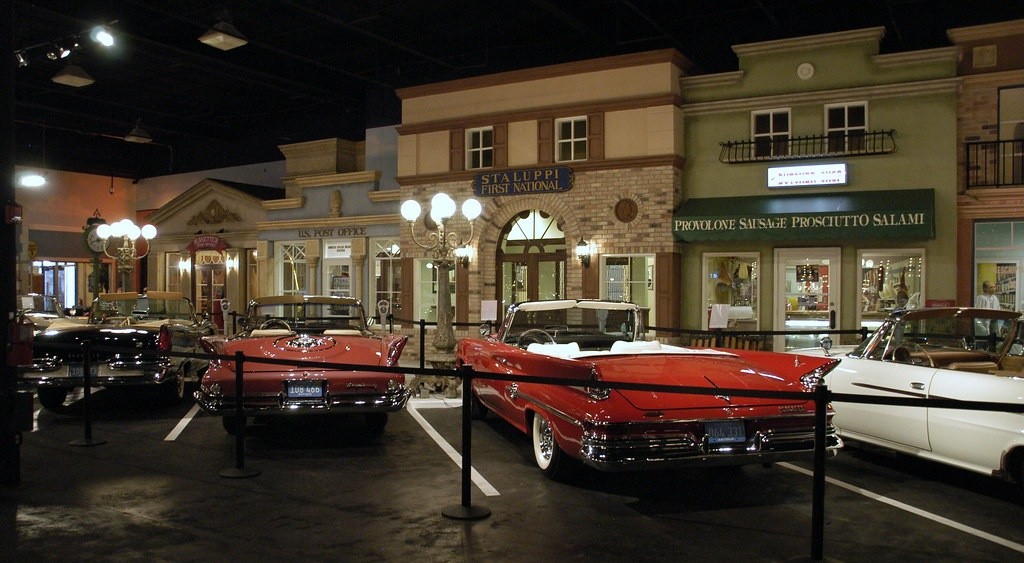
[787,307,1024,485]
[458,299,845,478]
[15,291,218,407]
[17,294,65,329]
[194,295,412,432]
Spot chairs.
[527,342,580,355]
[323,329,362,335]
[610,341,662,352]
[250,329,291,336]
[949,362,997,374]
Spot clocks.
[81,210,110,256]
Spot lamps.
[45,35,81,61]
[123,112,152,144]
[180,260,185,271]
[14,51,30,69]
[456,238,468,269]
[49,52,96,88]
[576,235,590,269]
[228,257,234,269]
[196,8,248,54]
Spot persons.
[975,280,1004,334]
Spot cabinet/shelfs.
[995,273,1016,311]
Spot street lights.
[96,219,157,293]
[401,193,482,353]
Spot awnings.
[671,188,936,243]
